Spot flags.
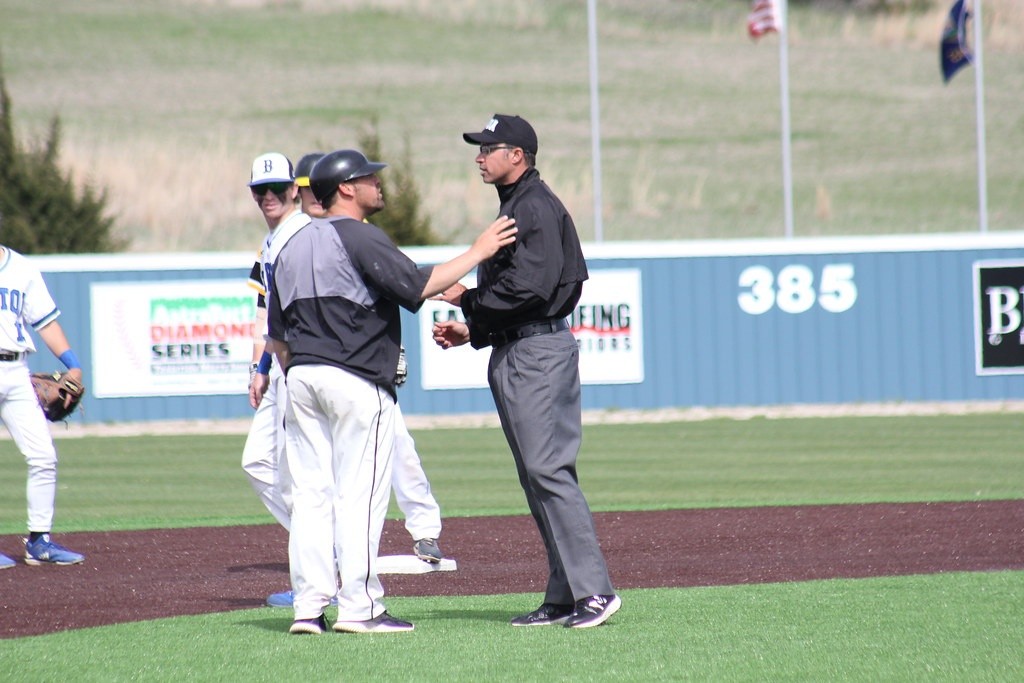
[749,0,787,37]
[939,0,980,83]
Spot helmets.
[309,150,387,205]
[294,154,326,187]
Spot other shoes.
[267,591,337,608]
[413,537,441,563]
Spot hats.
[463,113,537,155]
[247,153,295,186]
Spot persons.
[240,147,443,607]
[429,113,622,628]
[267,151,518,636]
[0,242,86,570]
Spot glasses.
[480,146,513,156]
[250,182,293,196]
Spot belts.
[488,318,569,348]
[0,351,29,361]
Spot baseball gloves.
[29,370,86,432]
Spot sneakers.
[562,594,622,628]
[21,534,85,567]
[510,603,577,627]
[290,613,327,634]
[0,553,16,570]
[332,610,414,633]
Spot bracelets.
[256,350,272,375]
[248,362,261,389]
[59,349,81,369]
